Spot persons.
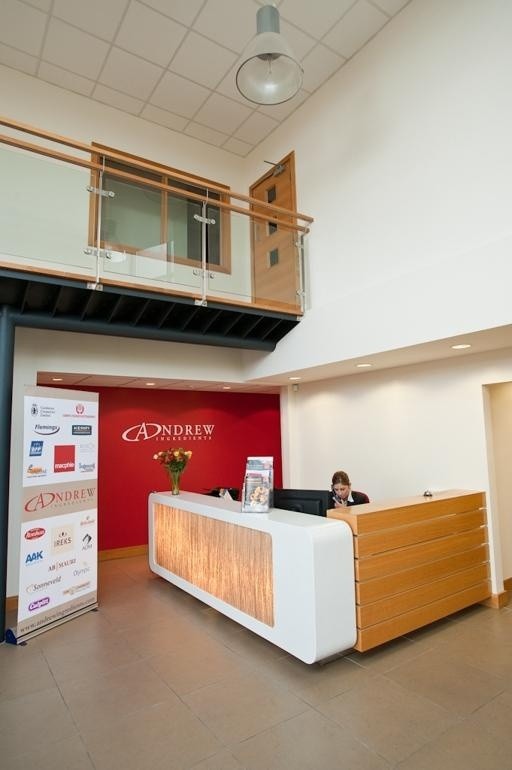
[330,471,369,509]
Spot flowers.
[152,448,193,491]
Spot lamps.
[235,1,306,108]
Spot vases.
[164,465,184,494]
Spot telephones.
[331,485,341,503]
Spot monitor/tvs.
[206,487,239,501]
[274,489,331,517]
